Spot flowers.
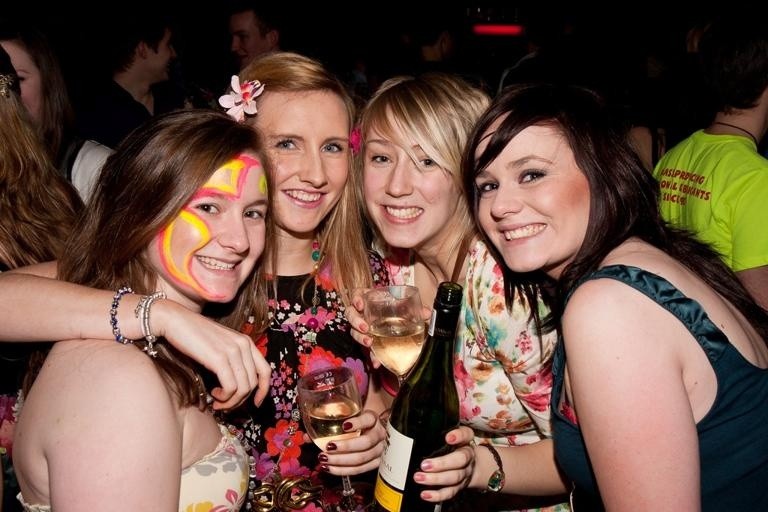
[214,72,265,121]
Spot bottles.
[374,281,463,511]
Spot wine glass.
[297,367,367,510]
[363,286,424,394]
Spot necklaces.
[192,371,256,512]
[255,233,321,482]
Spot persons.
[0,46,85,512]
[1,0,767,322]
[320,73,574,510]
[12,110,274,512]
[0,51,393,511]
[455,76,768,511]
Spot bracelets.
[480,438,504,496]
[110,286,137,344]
[134,291,171,358]
